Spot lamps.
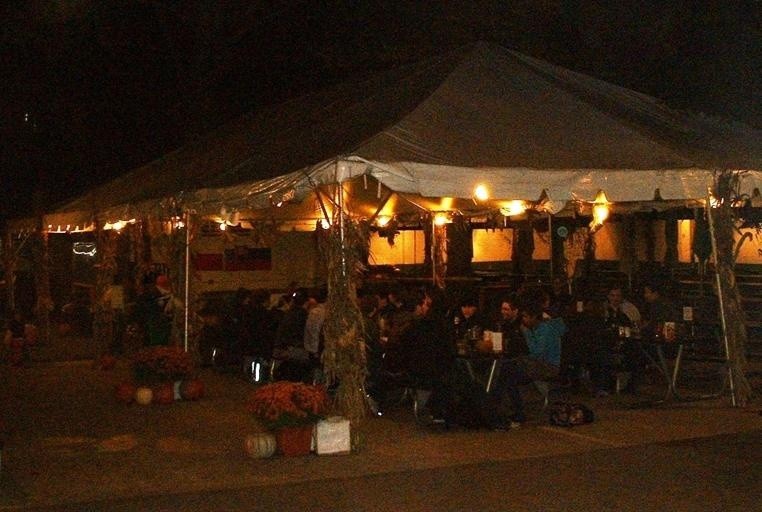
[586,186,616,227]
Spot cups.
[470,325,480,340]
[456,341,467,357]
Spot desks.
[443,345,529,393]
[614,329,727,408]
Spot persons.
[2,265,175,354]
[201,268,676,431]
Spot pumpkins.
[114,379,205,406]
[243,427,277,459]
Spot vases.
[273,429,311,458]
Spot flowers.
[130,343,195,380]
[244,378,328,426]
